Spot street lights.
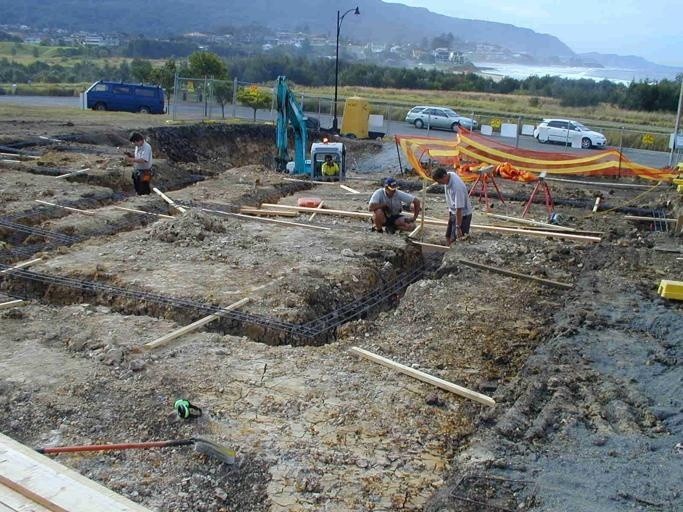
[329,6,360,134]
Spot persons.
[368,177,420,233]
[321,154,340,182]
[431,166,473,244]
[124,132,153,195]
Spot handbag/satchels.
[140,169,151,182]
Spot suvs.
[534,118,607,149]
[405,105,478,132]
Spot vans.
[84,80,170,115]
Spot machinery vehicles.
[273,75,346,182]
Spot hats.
[385,177,399,191]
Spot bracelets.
[456,225,461,228]
